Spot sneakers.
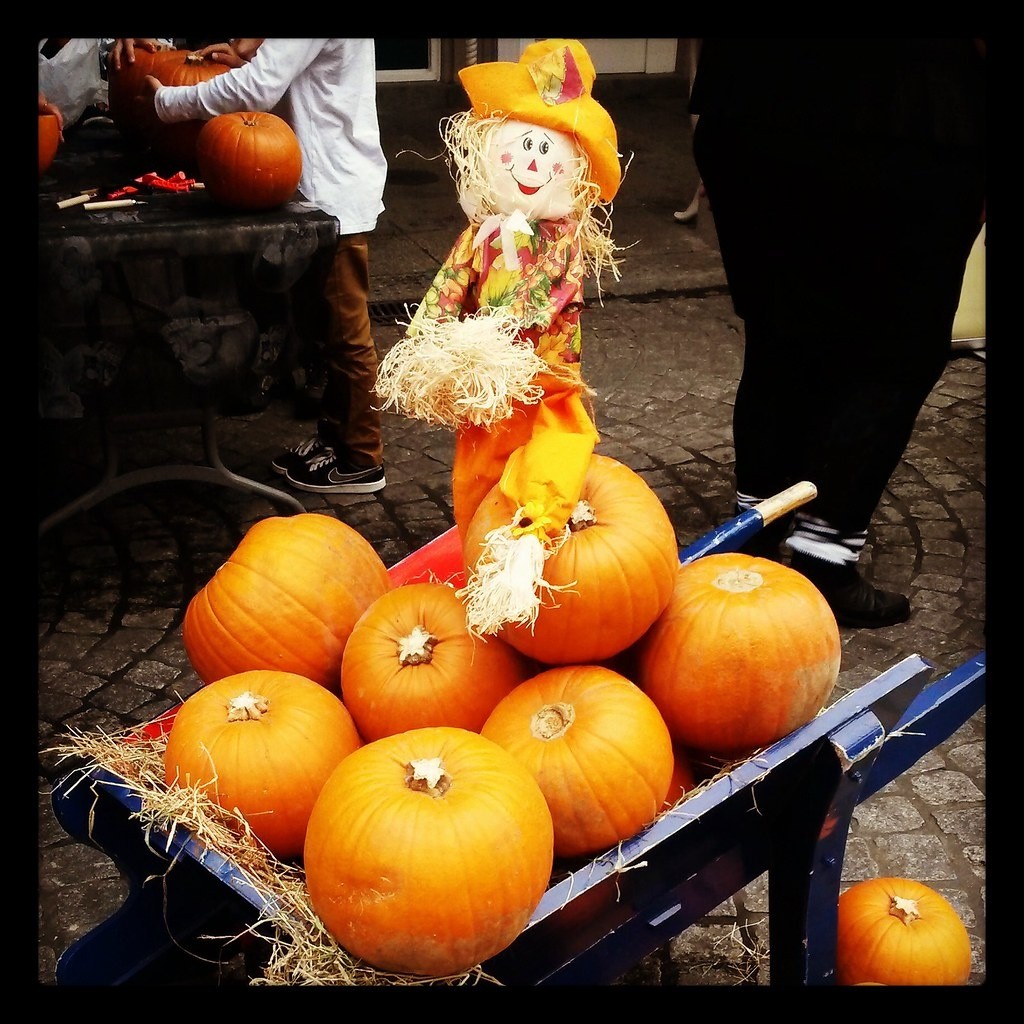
[286,451,388,493]
[272,434,339,475]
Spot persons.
[138,37,394,498]
[106,39,268,72]
[38,92,67,146]
[358,36,628,636]
[683,36,986,635]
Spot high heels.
[674,178,703,224]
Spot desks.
[35,114,345,574]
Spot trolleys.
[50,482,984,982]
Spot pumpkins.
[39,40,304,212]
[166,444,977,985]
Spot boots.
[785,509,912,629]
[729,485,790,560]
